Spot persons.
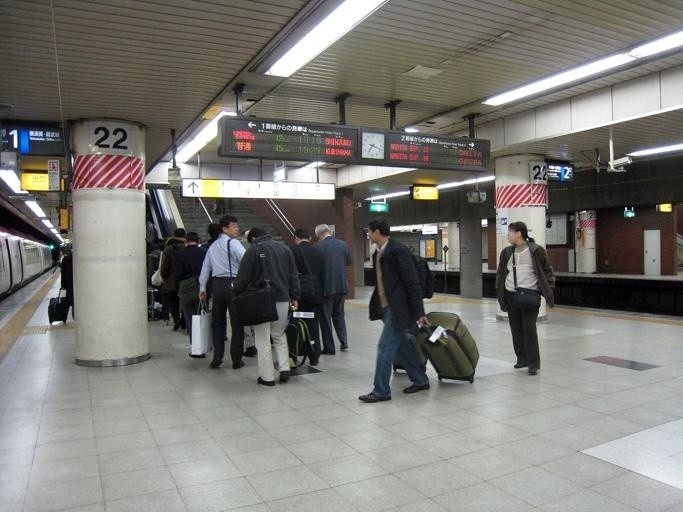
[358,217,430,403]
[145,197,353,386]
[51,242,74,324]
[495,222,556,374]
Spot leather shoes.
[527,368,537,374]
[279,371,290,383]
[233,359,245,369]
[173,318,182,330]
[359,392,391,402]
[243,346,257,356]
[210,357,223,368]
[513,363,528,368]
[258,377,275,386]
[403,383,429,393]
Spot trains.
[0,229,54,299]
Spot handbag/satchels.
[234,243,279,325]
[149,302,163,321]
[191,296,214,356]
[284,305,315,358]
[151,269,163,287]
[297,246,324,306]
[514,288,541,310]
[179,274,199,306]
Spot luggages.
[416,311,478,384]
[48,287,70,323]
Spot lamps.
[253,1,386,79]
[622,29,681,64]
[477,51,638,110]
[0,150,29,193]
[25,197,68,245]
[171,109,237,164]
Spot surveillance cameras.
[607,156,633,169]
[352,202,362,208]
[465,191,487,204]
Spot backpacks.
[169,243,187,275]
[410,256,435,298]
